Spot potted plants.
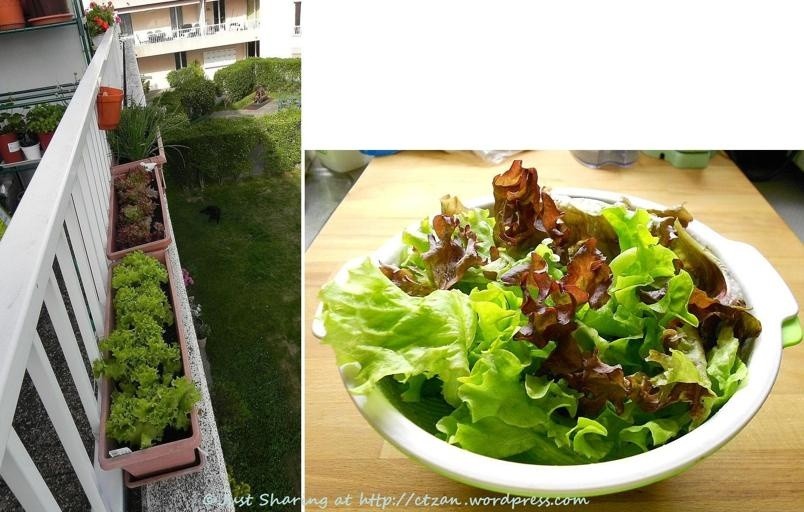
[92,85,206,491]
[0,97,70,167]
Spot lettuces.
[318,160,748,463]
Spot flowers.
[81,0,124,37]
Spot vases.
[0,0,74,31]
